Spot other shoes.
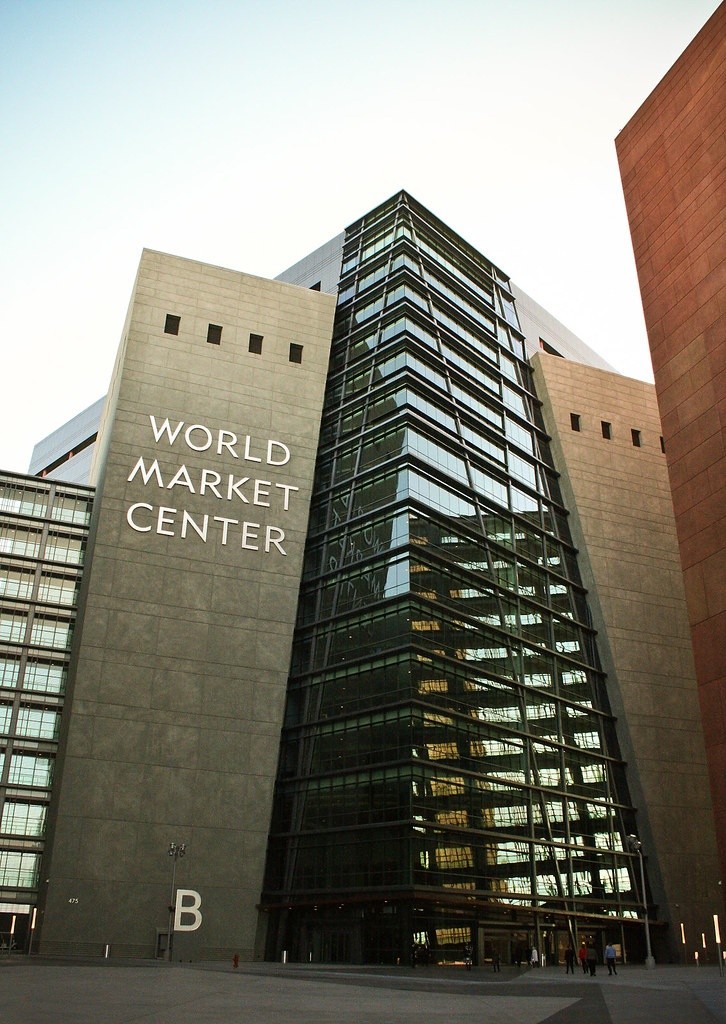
[593,974,596,976]
[615,972,617,975]
[608,973,612,975]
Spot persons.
[515,944,523,966]
[564,945,574,974]
[411,943,417,968]
[585,944,598,976]
[605,943,617,975]
[492,950,501,972]
[531,947,540,968]
[464,950,472,970]
[525,945,531,966]
[579,945,588,974]
